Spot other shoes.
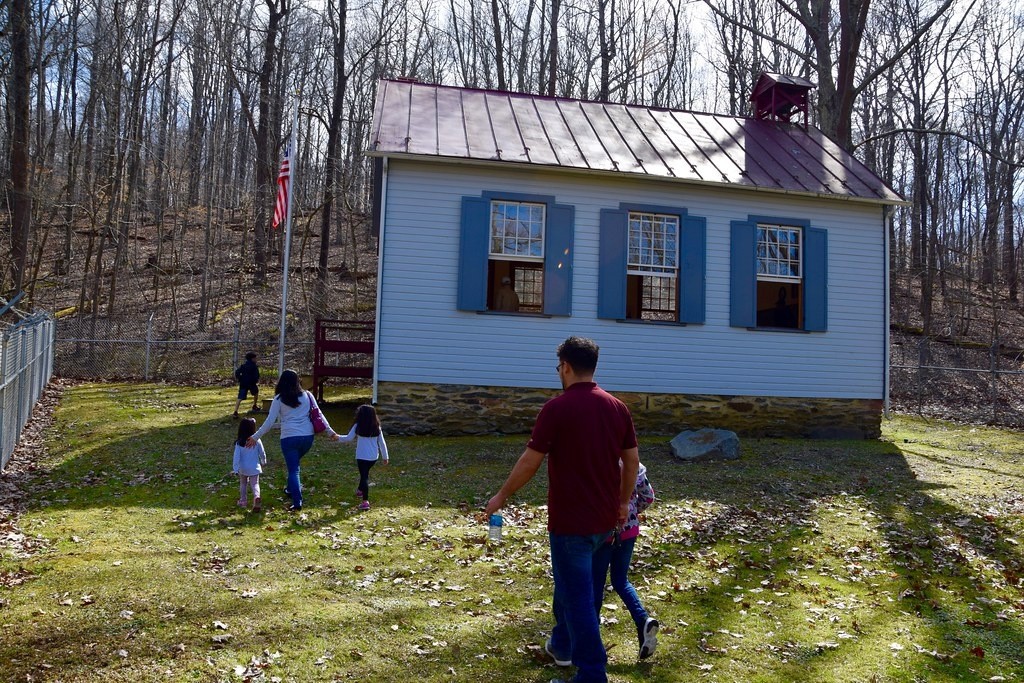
[284,488,290,496]
[288,504,301,511]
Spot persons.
[332,404,389,509]
[591,450,659,658]
[232,418,267,512]
[246,370,339,510]
[231,352,261,416]
[496,276,520,312]
[473,335,640,683]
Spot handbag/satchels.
[306,390,326,433]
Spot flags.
[274,137,291,225]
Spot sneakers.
[252,405,261,411]
[544,638,573,666]
[357,501,370,510]
[237,499,247,508]
[356,489,363,497]
[232,413,239,418]
[636,618,660,660]
[253,496,262,513]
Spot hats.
[501,276,511,285]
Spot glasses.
[555,362,563,372]
